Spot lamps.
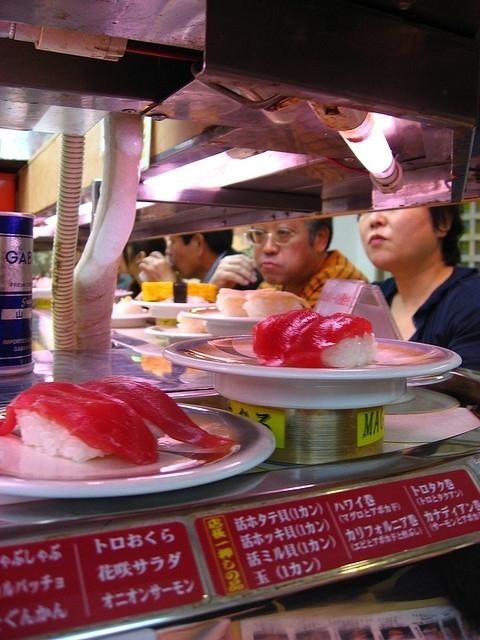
[308,95,405,193]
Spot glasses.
[246,227,301,243]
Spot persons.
[116,254,134,291]
[117,237,169,301]
[354,204,480,372]
[206,215,364,315]
[136,229,263,306]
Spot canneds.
[0,210,36,292]
[1,294,34,376]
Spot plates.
[162,335,463,409]
[0,404,276,499]
[110,277,308,335]
